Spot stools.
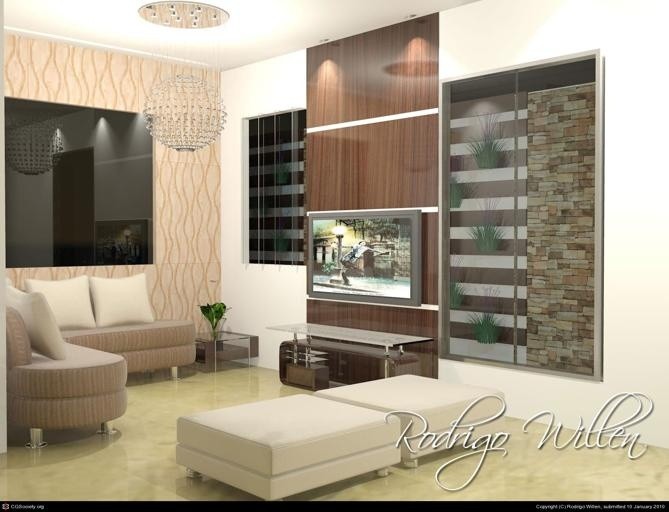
[174,372,506,501]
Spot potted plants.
[199,300,228,338]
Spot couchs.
[0,272,198,451]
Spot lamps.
[7,114,66,174]
[135,1,230,33]
[143,67,230,155]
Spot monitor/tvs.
[93,218,153,265]
[309,209,422,307]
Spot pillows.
[4,271,153,362]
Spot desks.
[266,320,435,389]
